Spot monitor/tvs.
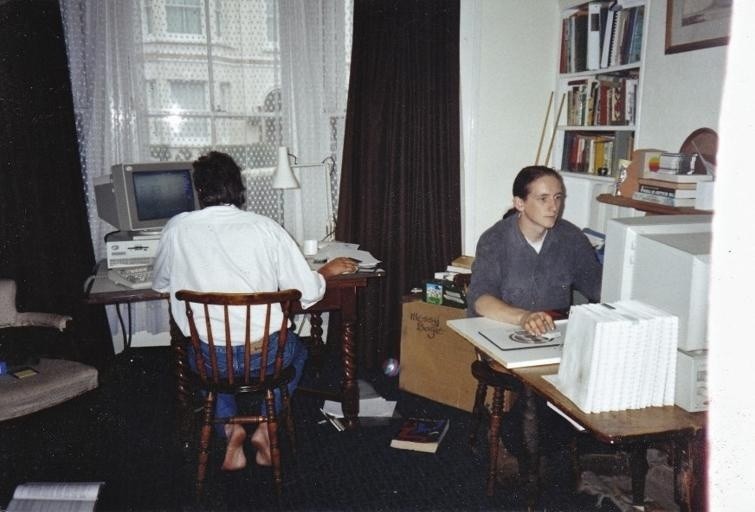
[91,160,202,239]
[599,213,711,350]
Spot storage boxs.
[397,298,481,414]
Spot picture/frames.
[663,1,728,56]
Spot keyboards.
[104,265,152,290]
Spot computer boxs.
[673,351,707,413]
[106,233,161,268]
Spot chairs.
[453,274,585,499]
[164,289,303,502]
[0,279,98,478]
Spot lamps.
[275,146,335,249]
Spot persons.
[152,151,360,471]
[466,166,602,496]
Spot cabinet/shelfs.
[555,1,652,231]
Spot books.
[390,416,450,454]
[561,129,635,178]
[320,379,397,433]
[632,154,713,207]
[434,254,476,309]
[555,299,680,415]
[6,480,106,512]
[559,0,645,74]
[567,71,638,126]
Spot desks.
[448,317,708,512]
[85,256,387,431]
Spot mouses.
[342,265,358,275]
[540,324,561,338]
